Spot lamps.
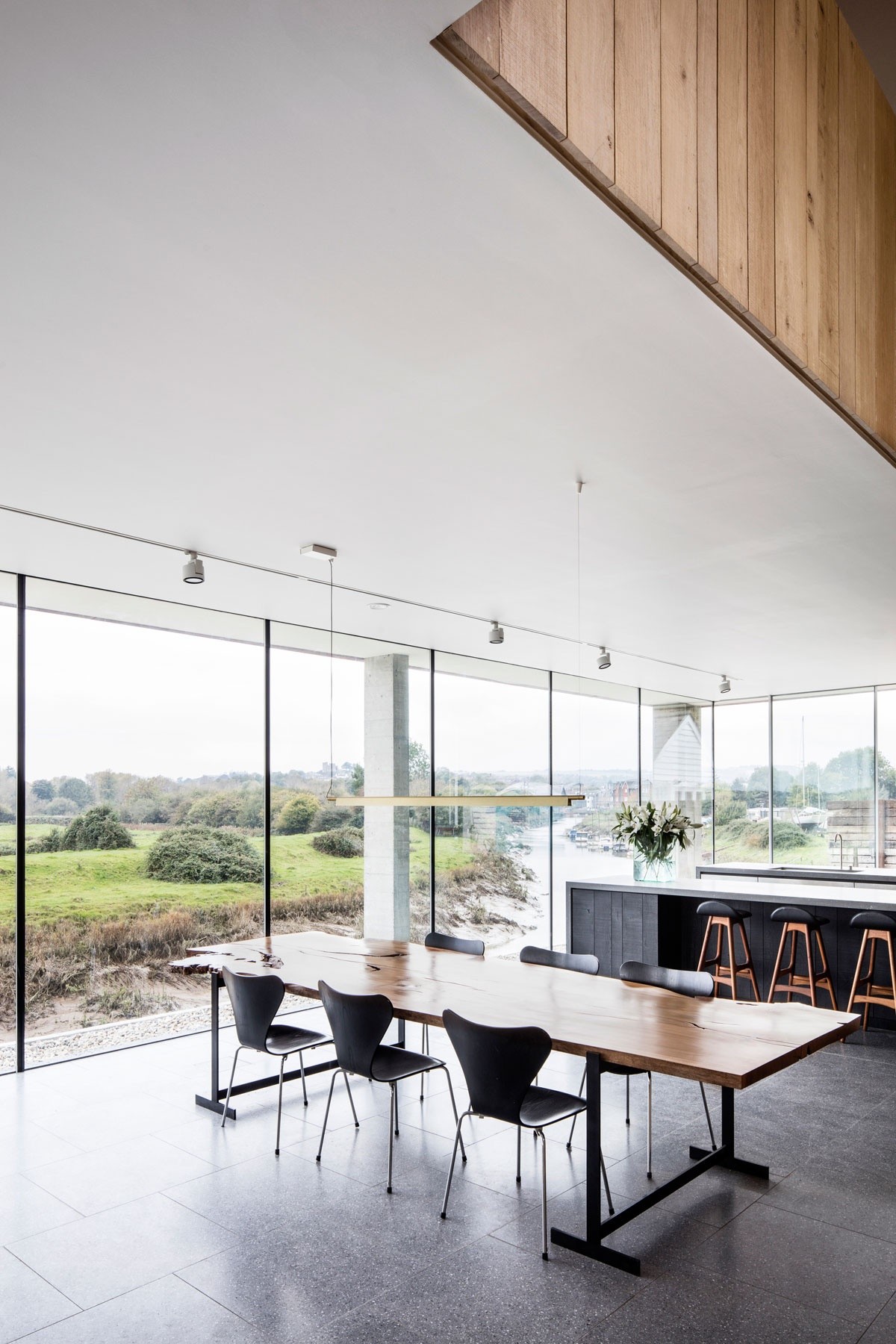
[182,551,204,584]
[719,674,731,693]
[596,646,611,669]
[487,622,505,644]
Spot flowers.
[617,802,704,880]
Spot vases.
[633,845,676,883]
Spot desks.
[166,930,862,1267]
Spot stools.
[697,901,761,1001]
[766,907,838,1013]
[839,911,896,1043]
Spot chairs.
[504,946,600,1134]
[567,963,714,1179]
[416,931,486,1099]
[220,965,360,1156]
[313,980,467,1195]
[441,1009,616,1260]
